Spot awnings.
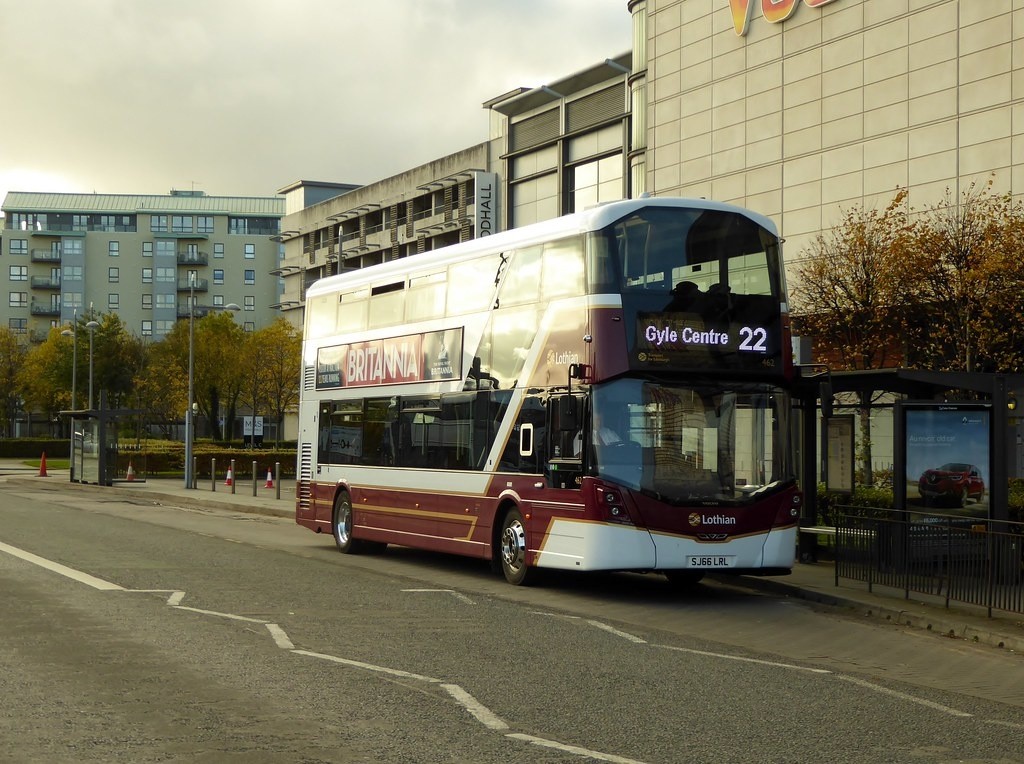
[805,362,998,402]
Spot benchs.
[798,516,877,564]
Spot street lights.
[86,301,100,409]
[60,309,77,468]
[184,274,241,489]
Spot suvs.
[918,463,985,508]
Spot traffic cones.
[225,465,232,486]
[34,452,52,477]
[264,466,275,488]
[126,461,134,480]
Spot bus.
[295,193,835,587]
